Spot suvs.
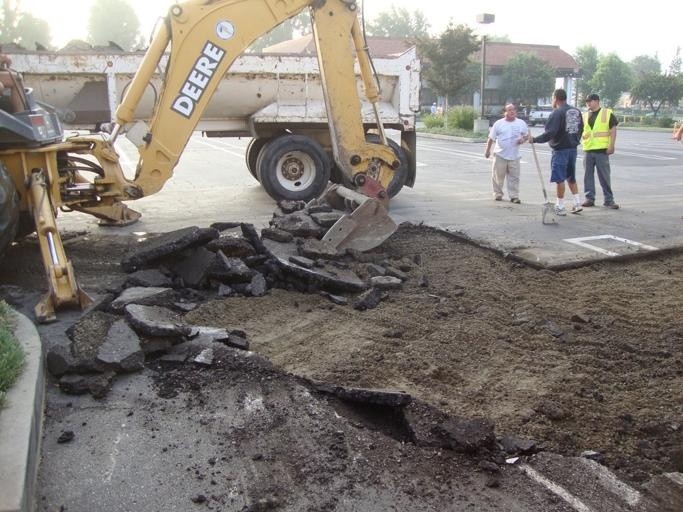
[515,104,552,127]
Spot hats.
[584,93,599,100]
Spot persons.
[579,93,620,210]
[528,88,584,216]
[437,103,443,114]
[484,101,531,203]
[430,101,436,114]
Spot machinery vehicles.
[0,0,402,324]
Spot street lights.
[474,13,494,134]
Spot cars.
[645,112,660,117]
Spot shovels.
[528,128,558,224]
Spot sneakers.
[510,198,521,204]
[603,200,619,209]
[569,202,584,213]
[495,195,502,201]
[581,198,594,207]
[555,204,566,216]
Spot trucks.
[0,48,418,206]
